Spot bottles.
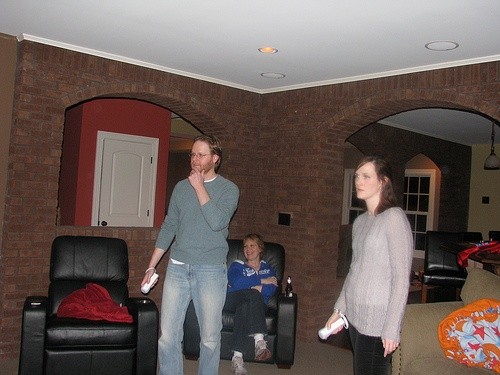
[284,276,293,297]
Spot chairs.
[421,230,482,304]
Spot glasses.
[191,153,212,158]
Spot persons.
[141,135,240,375]
[224,233,278,375]
[325,155,414,375]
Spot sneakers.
[255,340,271,361]
[230,356,248,375]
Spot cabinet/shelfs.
[92,131,159,227]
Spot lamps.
[485,121,500,170]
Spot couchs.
[183,240,298,370]
[391,268,500,375]
[18,235,159,375]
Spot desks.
[470,251,500,276]
